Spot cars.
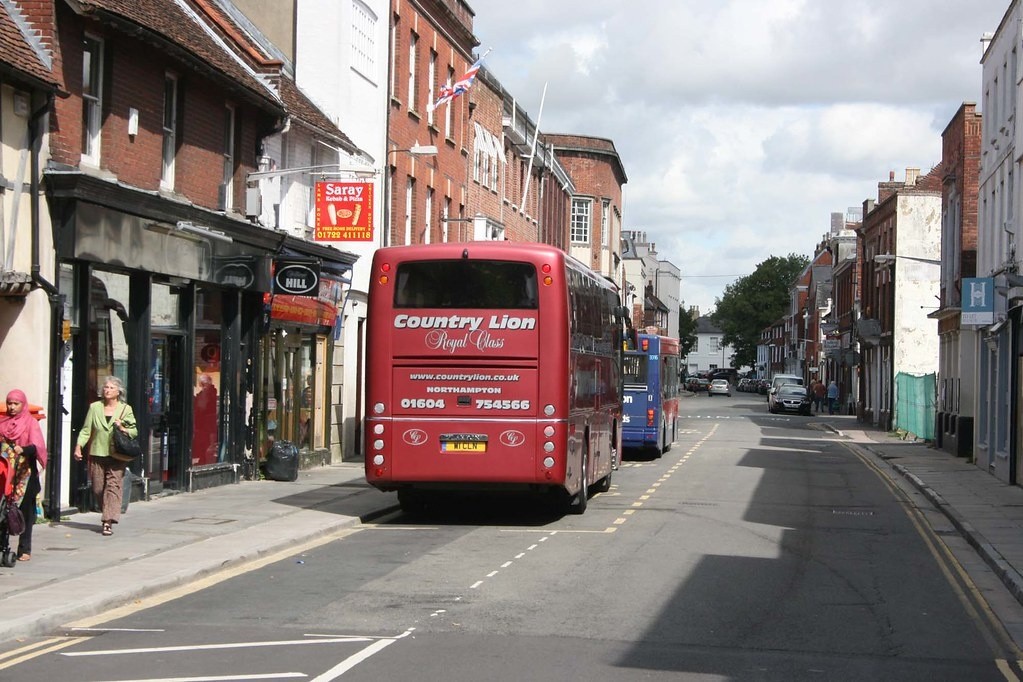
[684,366,813,417]
[706,378,731,397]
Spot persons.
[194,375,217,464]
[74,377,139,536]
[813,380,826,413]
[810,379,817,403]
[827,381,838,415]
[0,390,48,561]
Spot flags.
[435,56,485,108]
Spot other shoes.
[102,521,113,536]
[17,552,31,561]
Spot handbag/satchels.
[111,420,143,458]
[8,505,26,537]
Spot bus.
[620,333,680,458]
[362,238,639,515]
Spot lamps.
[388,146,438,156]
[803,315,840,322]
[874,255,942,265]
[177,221,233,244]
[308,171,377,178]
[769,344,786,347]
